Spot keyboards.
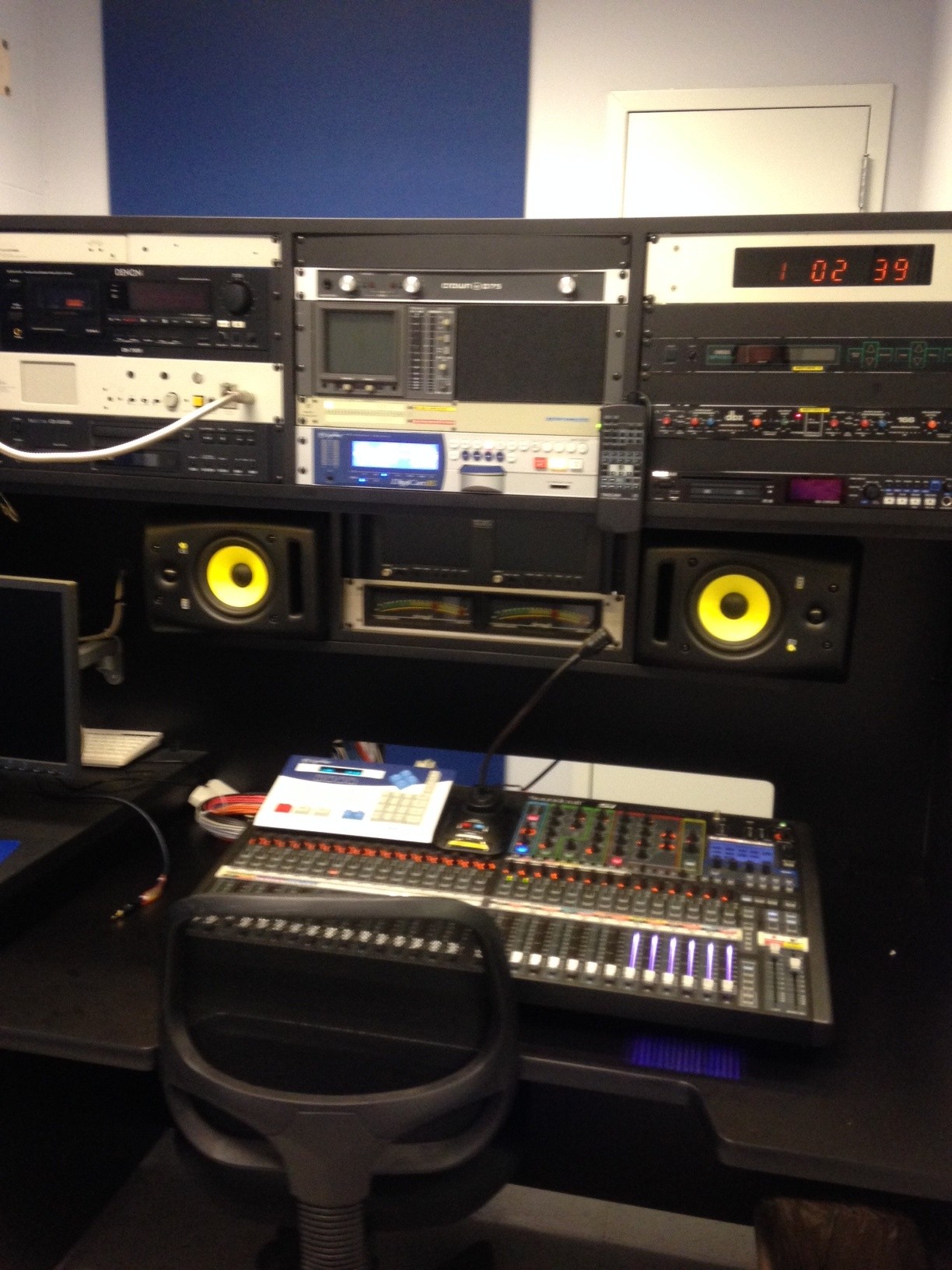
[80,727,163,767]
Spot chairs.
[150,896,521,1270]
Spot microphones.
[436,629,611,854]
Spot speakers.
[637,539,856,678]
[142,517,323,639]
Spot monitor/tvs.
[0,575,81,782]
